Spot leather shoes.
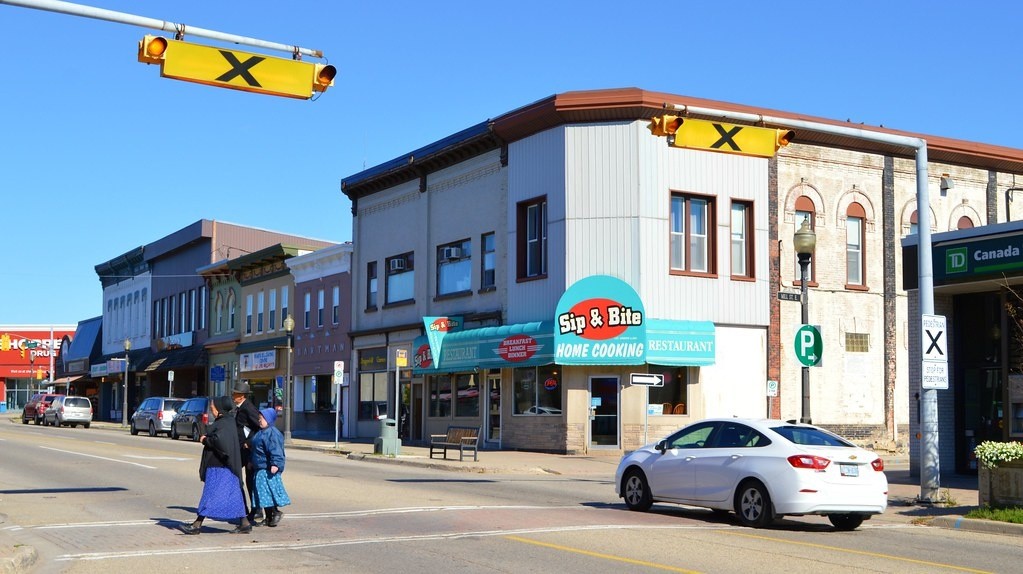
[256,519,269,527]
[246,513,263,521]
[270,511,282,525]
[229,525,251,534]
[179,525,200,534]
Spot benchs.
[430,423,482,460]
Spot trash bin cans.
[374,418,398,455]
[0,402,7,413]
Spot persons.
[252,408,285,527]
[178,396,252,534]
[231,382,262,524]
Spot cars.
[43,396,93,428]
[22,394,64,425]
[130,396,189,437]
[170,396,215,441]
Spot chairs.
[721,429,740,443]
[662,402,685,414]
[773,428,794,442]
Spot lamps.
[990,320,1000,340]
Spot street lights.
[283,313,295,439]
[30,352,35,399]
[123,339,131,426]
[793,214,816,426]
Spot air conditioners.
[445,248,460,258]
[391,259,404,270]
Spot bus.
[614,416,887,531]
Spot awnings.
[135,344,209,372]
[46,376,83,386]
[410,275,714,375]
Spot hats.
[259,408,277,426]
[229,382,253,394]
[212,396,233,414]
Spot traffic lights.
[651,115,684,136]
[313,63,338,93]
[138,35,168,65]
[774,128,796,151]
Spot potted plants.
[975,441,1023,509]
